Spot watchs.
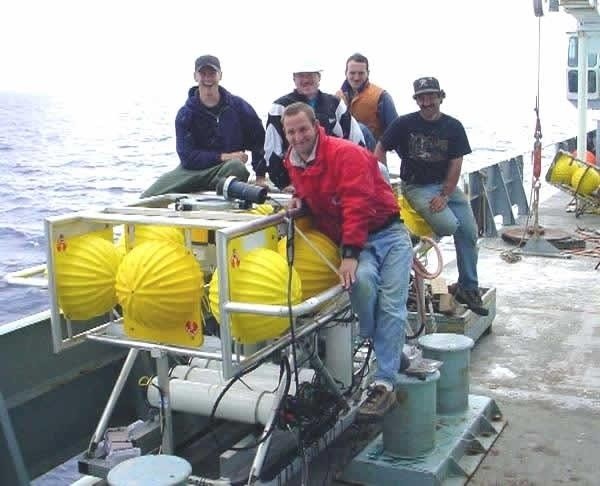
[439,192,449,201]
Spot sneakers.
[399,352,410,371]
[455,286,489,316]
[355,385,399,424]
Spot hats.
[413,77,440,94]
[291,61,324,74]
[195,55,220,72]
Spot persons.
[335,52,400,168]
[280,100,415,418]
[262,61,368,196]
[139,54,267,200]
[373,77,489,317]
